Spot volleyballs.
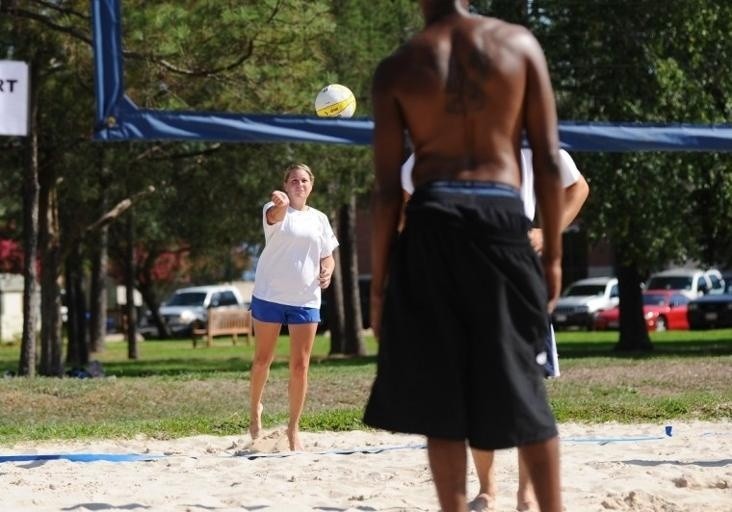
[315,84,357,119]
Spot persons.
[246,162,340,452]
[363,0,568,512]
[395,148,593,512]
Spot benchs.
[191,306,255,350]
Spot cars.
[553,268,732,332]
[137,285,246,340]
